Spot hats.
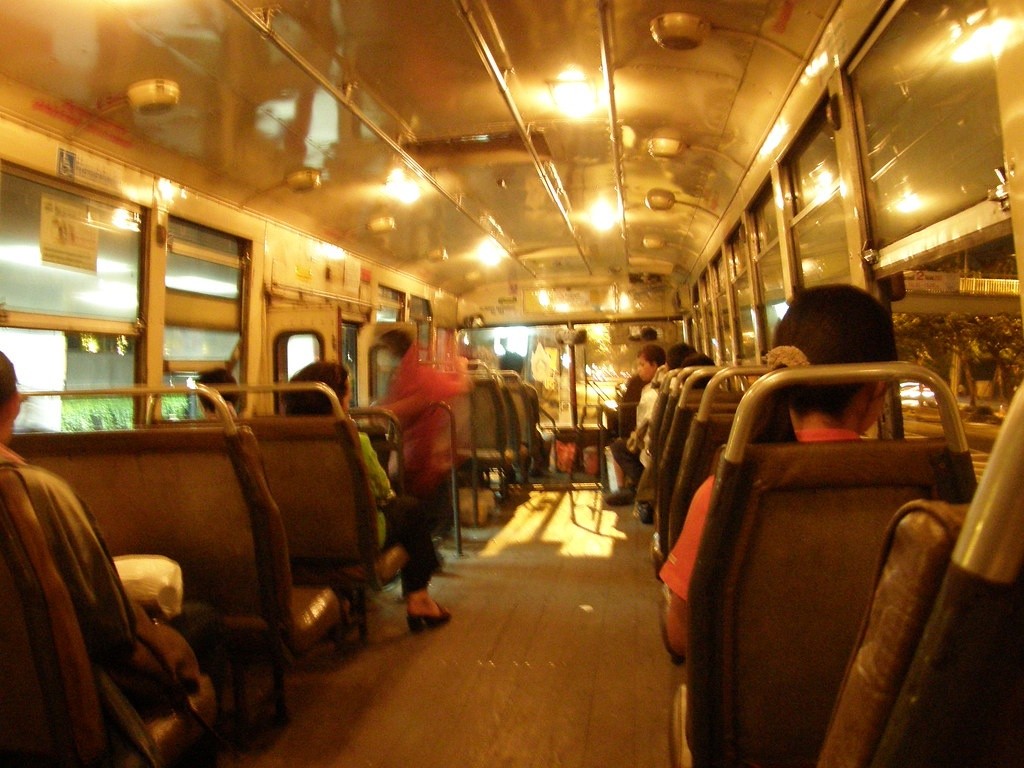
[370,327,414,352]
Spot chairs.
[618,359,1024,768]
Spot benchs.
[0,357,544,768]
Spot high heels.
[407,603,451,633]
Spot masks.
[376,351,401,371]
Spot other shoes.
[606,486,637,506]
[632,500,655,523]
